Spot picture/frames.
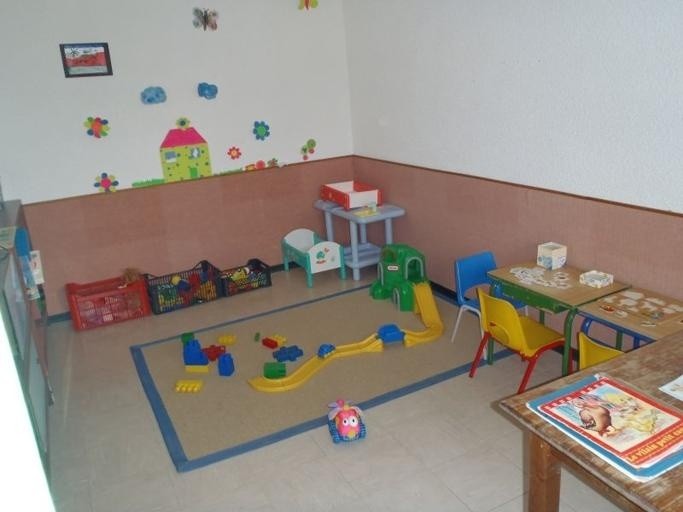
[58,42,114,78]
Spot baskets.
[65,258,272,331]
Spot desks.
[486,327,682,510]
[486,260,635,378]
[575,286,683,351]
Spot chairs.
[449,251,575,394]
[579,332,626,371]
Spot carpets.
[128,283,518,474]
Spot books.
[524,372,682,484]
[26,249,45,286]
[13,225,35,272]
[13,255,40,304]
[537,377,682,470]
[0,225,15,252]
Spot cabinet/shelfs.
[0,200,51,485]
